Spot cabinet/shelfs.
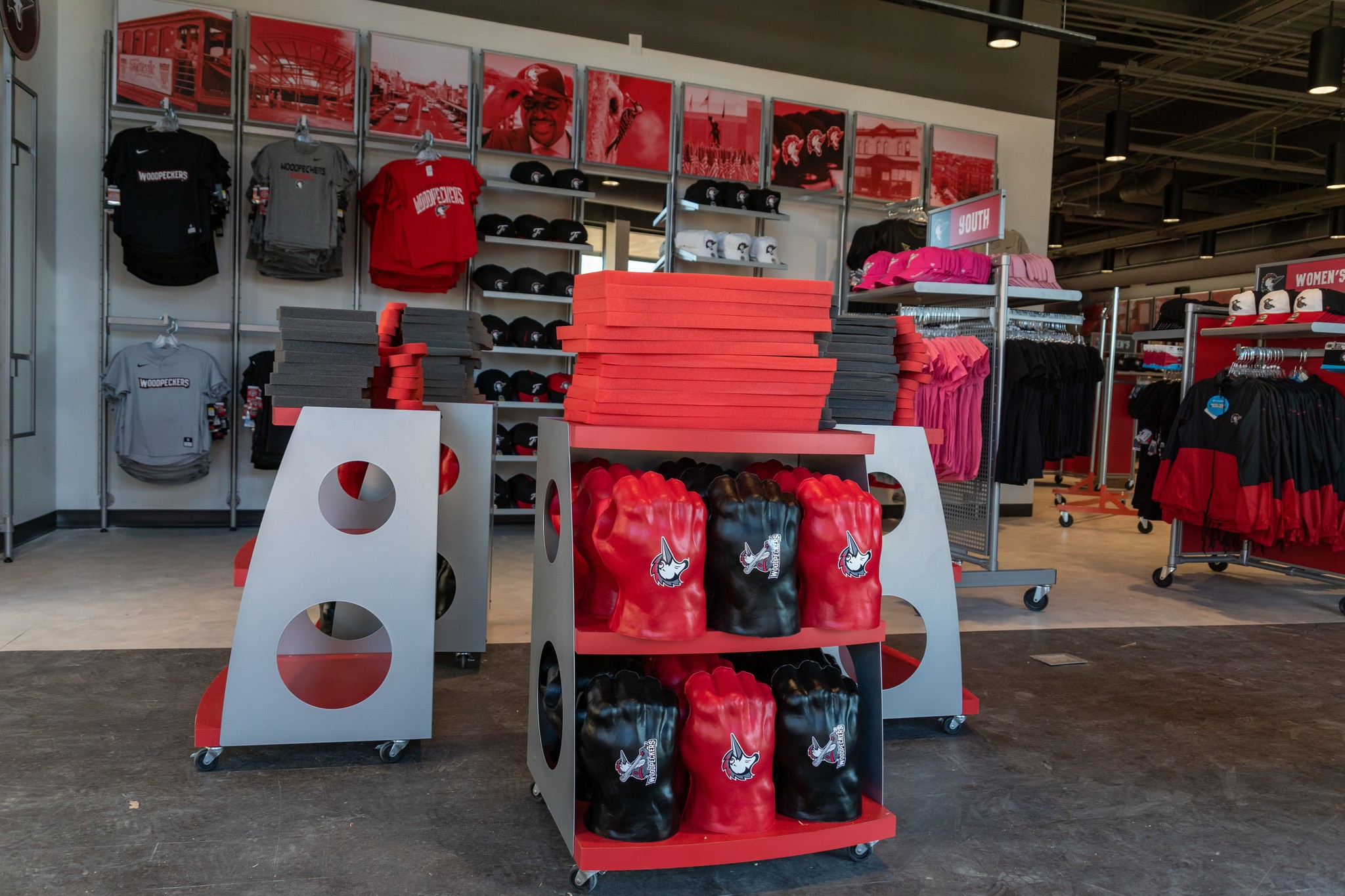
[675,197,791,271]
[480,177,597,517]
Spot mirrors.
[580,170,673,278]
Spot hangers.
[155,315,179,349]
[1227,345,1312,382]
[898,305,1087,347]
[294,117,321,148]
[412,131,442,162]
[151,100,182,132]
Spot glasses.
[520,97,562,110]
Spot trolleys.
[524,415,898,894]
[189,405,440,772]
[1150,301,1345,617]
[846,253,1088,612]
[1043,285,1185,534]
[833,425,980,735]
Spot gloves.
[540,459,882,842]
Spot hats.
[674,229,780,265]
[472,264,574,508]
[685,179,781,216]
[1221,289,1345,328]
[510,161,552,186]
[990,252,1063,290]
[516,62,566,99]
[476,213,588,244]
[853,247,991,293]
[552,168,591,192]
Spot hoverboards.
[435,402,496,670]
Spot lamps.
[1307,0,1345,94]
[1162,158,1184,224]
[1104,73,1130,162]
[987,0,1023,50]
[1048,207,1065,249]
[1327,207,1345,239]
[1199,213,1217,259]
[1324,109,1345,190]
[1100,226,1116,274]
